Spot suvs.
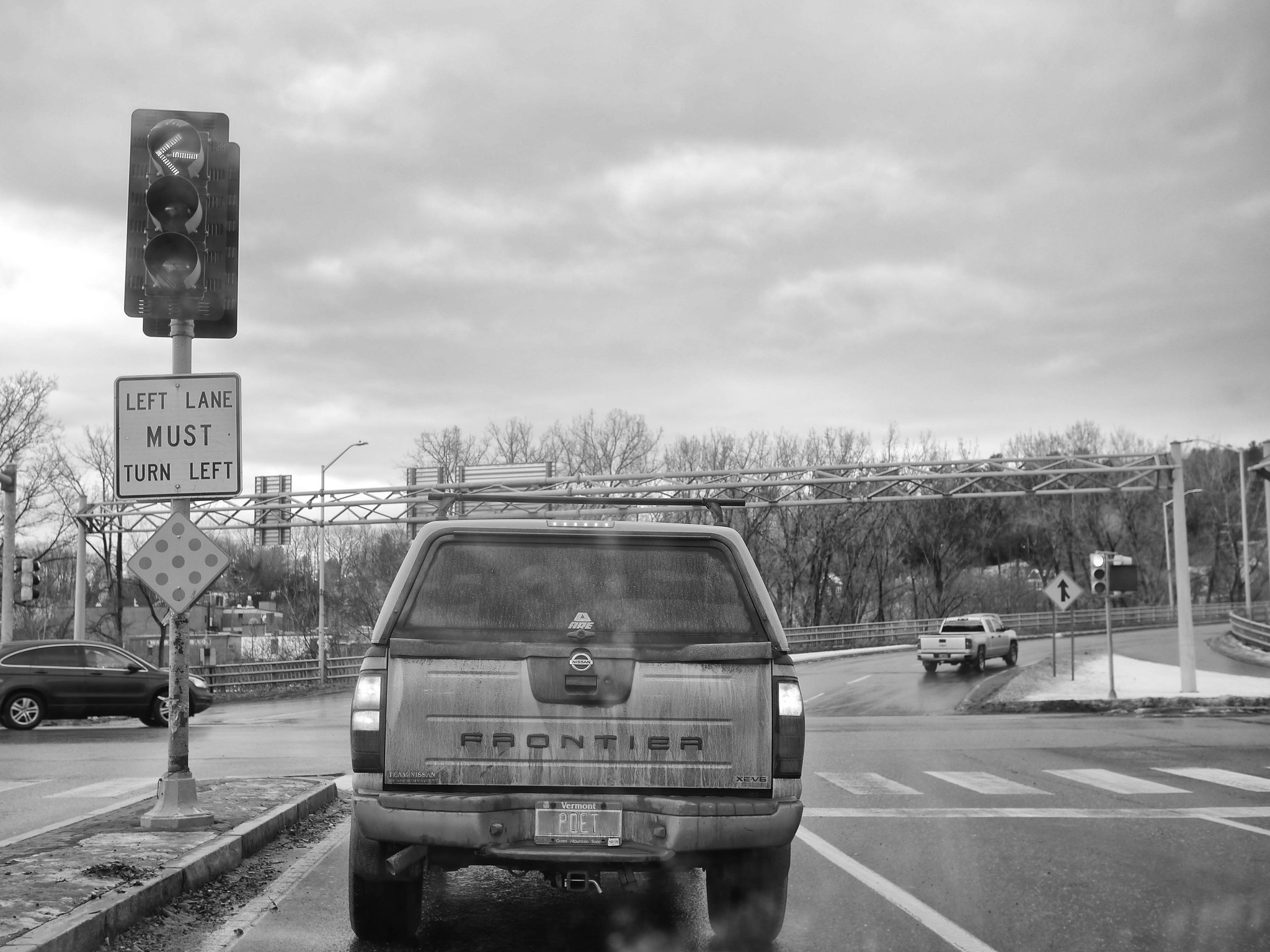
[347,517,807,941]
[0,639,214,730]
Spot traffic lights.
[124,108,229,322]
[1090,553,1107,596]
[20,558,40,601]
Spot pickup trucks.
[917,612,1018,672]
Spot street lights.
[318,441,369,685]
[1163,488,1203,605]
[1179,438,1252,620]
